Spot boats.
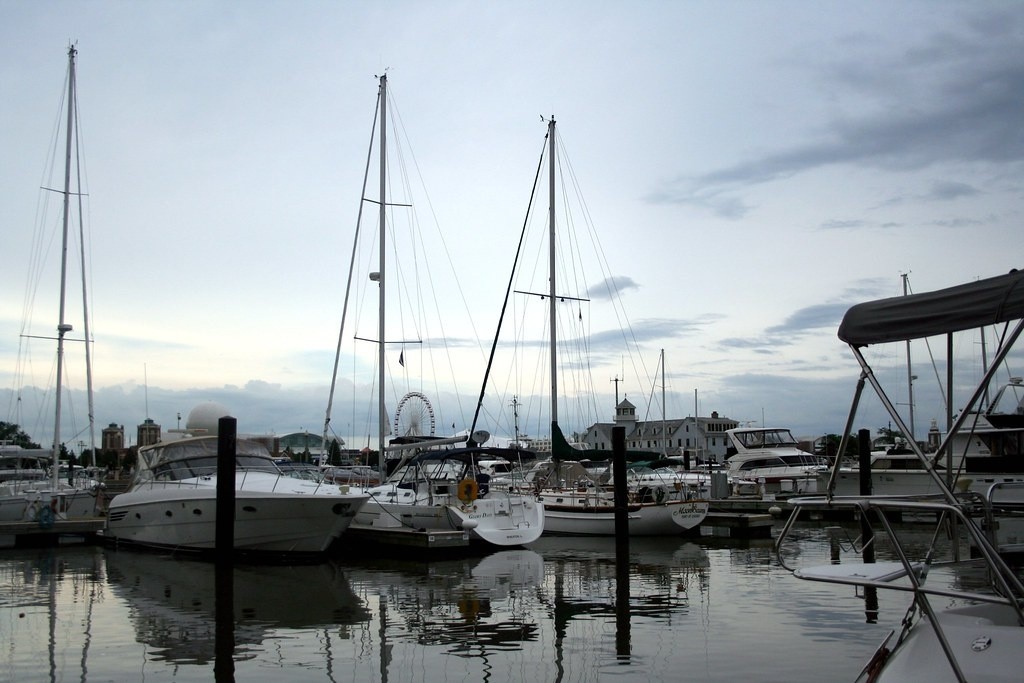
[776,267,1024,682]
[279,67,827,546]
[96,412,372,554]
[0,40,106,538]
